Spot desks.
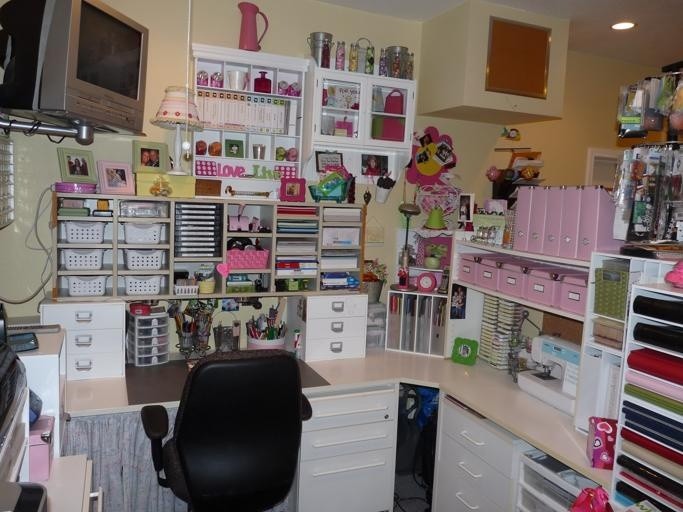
[54,319,616,512]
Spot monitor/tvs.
[38,0,149,132]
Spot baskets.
[225,249,269,268]
[63,221,163,294]
[174,286,199,295]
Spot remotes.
[6,324,60,337]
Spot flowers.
[422,241,447,258]
[359,258,388,283]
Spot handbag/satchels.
[384,91,403,113]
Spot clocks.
[416,272,436,293]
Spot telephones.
[438,266,450,294]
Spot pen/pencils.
[218,320,224,352]
[175,311,213,336]
[377,176,397,190]
[246,315,287,341]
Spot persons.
[141,149,159,166]
[66,154,88,177]
[450,287,465,318]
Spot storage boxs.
[134,170,199,203]
[512,183,624,263]
[456,247,589,318]
[226,242,270,269]
[367,302,387,329]
[366,328,386,349]
[127,308,170,370]
[27,412,56,482]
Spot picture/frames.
[94,158,136,197]
[313,149,344,172]
[276,176,307,203]
[221,138,243,158]
[130,138,169,174]
[457,193,473,223]
[56,145,100,185]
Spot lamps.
[388,201,419,292]
[149,84,207,178]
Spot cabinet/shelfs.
[570,246,682,441]
[384,226,453,359]
[439,225,591,415]
[188,41,309,187]
[308,55,420,157]
[45,189,367,301]
[35,294,131,388]
[279,292,371,364]
[35,447,105,512]
[11,325,74,463]
[608,275,683,511]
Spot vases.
[422,256,441,269]
[361,280,382,304]
